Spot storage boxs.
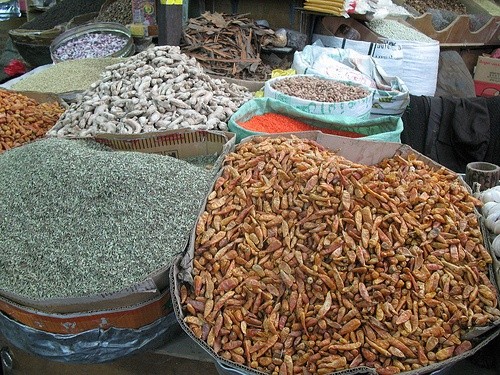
[0,0,500,375]
